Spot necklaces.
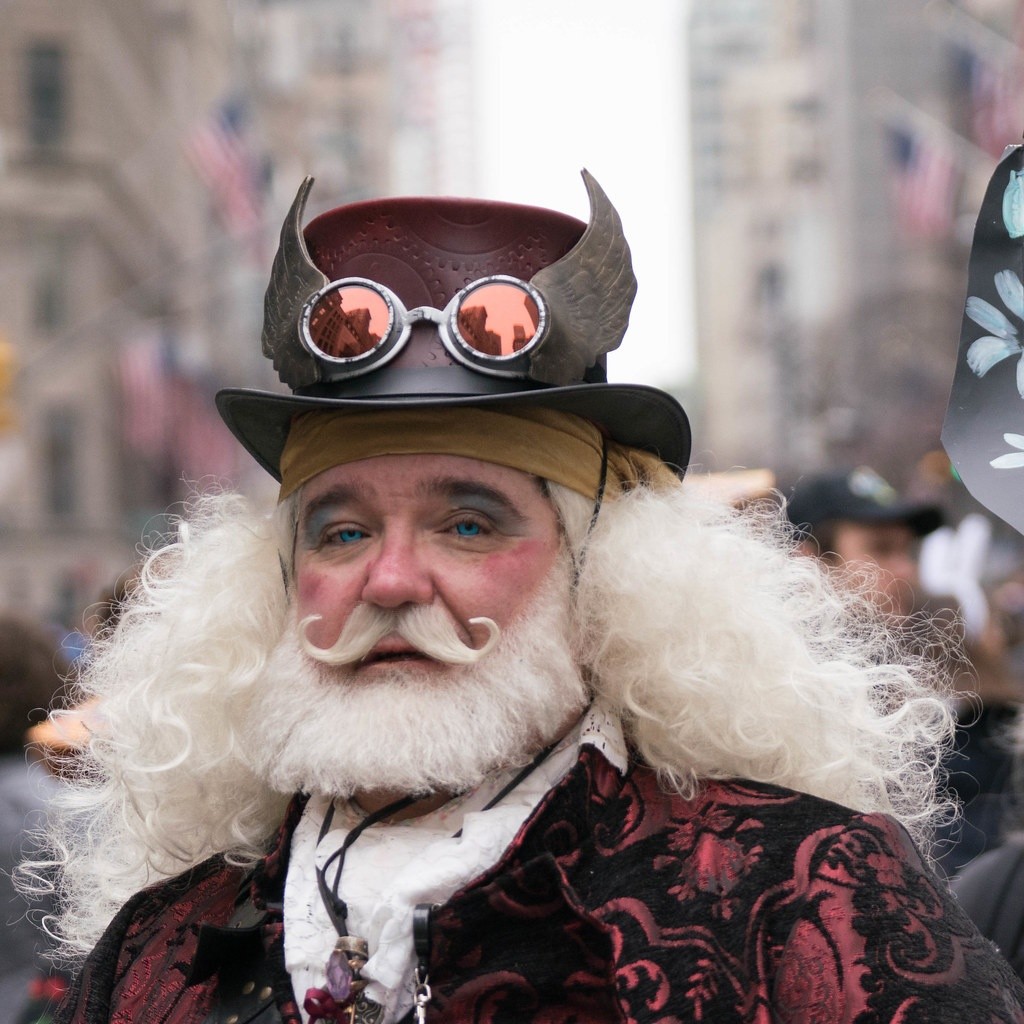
[302,787,431,1024]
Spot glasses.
[261,167,639,387]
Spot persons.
[31,168,1024,1024]
[0,463,1023,1024]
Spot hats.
[785,467,947,539]
[213,196,692,484]
